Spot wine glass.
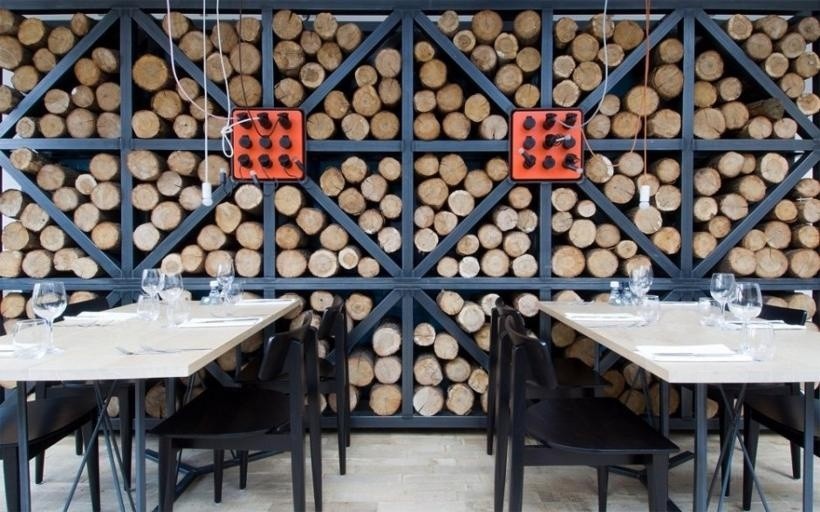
[728,279,764,354]
[628,264,655,323]
[710,271,736,330]
[140,267,164,322]
[30,281,69,355]
[157,270,183,324]
[217,262,235,293]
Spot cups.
[743,321,779,364]
[639,294,662,325]
[165,293,193,323]
[11,317,49,361]
[136,294,160,320]
[225,282,245,305]
[209,292,227,317]
[696,295,721,328]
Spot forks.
[112,343,211,356]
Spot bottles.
[209,280,219,295]
[608,279,636,308]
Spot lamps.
[640,1,651,210]
[200,1,215,209]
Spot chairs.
[494,316,678,510]
[0,395,106,512]
[740,383,820,510]
[483,298,615,457]
[34,297,151,491]
[675,305,809,487]
[148,307,325,512]
[230,297,349,486]
